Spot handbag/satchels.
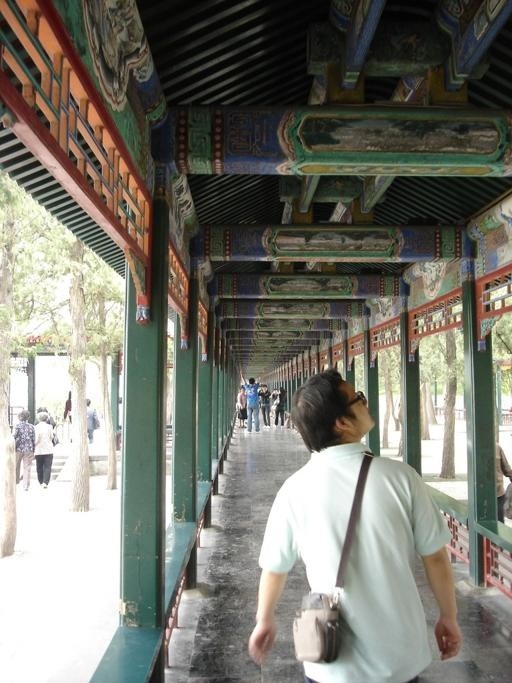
[284,588,341,666]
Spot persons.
[249,368,463,683]
[236,377,287,434]
[13,391,100,491]
[496,444,512,552]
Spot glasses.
[350,389,368,408]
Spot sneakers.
[238,424,285,433]
[22,483,48,493]
